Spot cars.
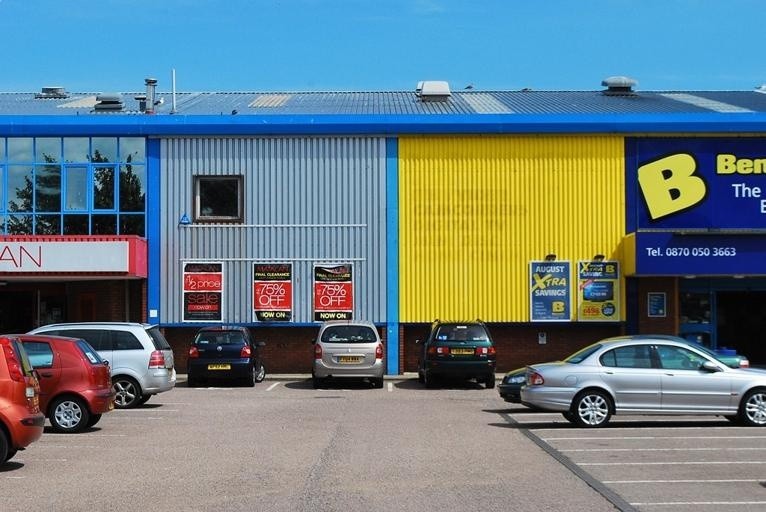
[187,324,265,386]
[0,322,177,467]
[417,319,496,389]
[311,319,386,389]
[498,331,766,426]
